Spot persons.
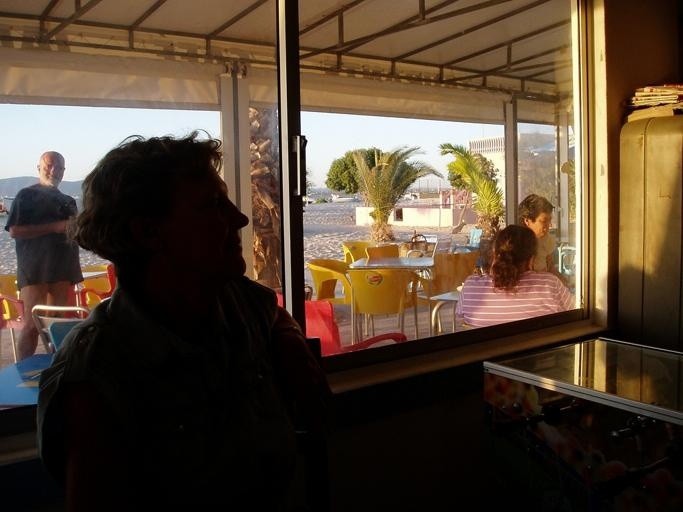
[453,223,576,331]
[516,193,574,292]
[31,128,375,511]
[3,151,84,360]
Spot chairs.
[0,264,117,354]
[305,229,484,357]
